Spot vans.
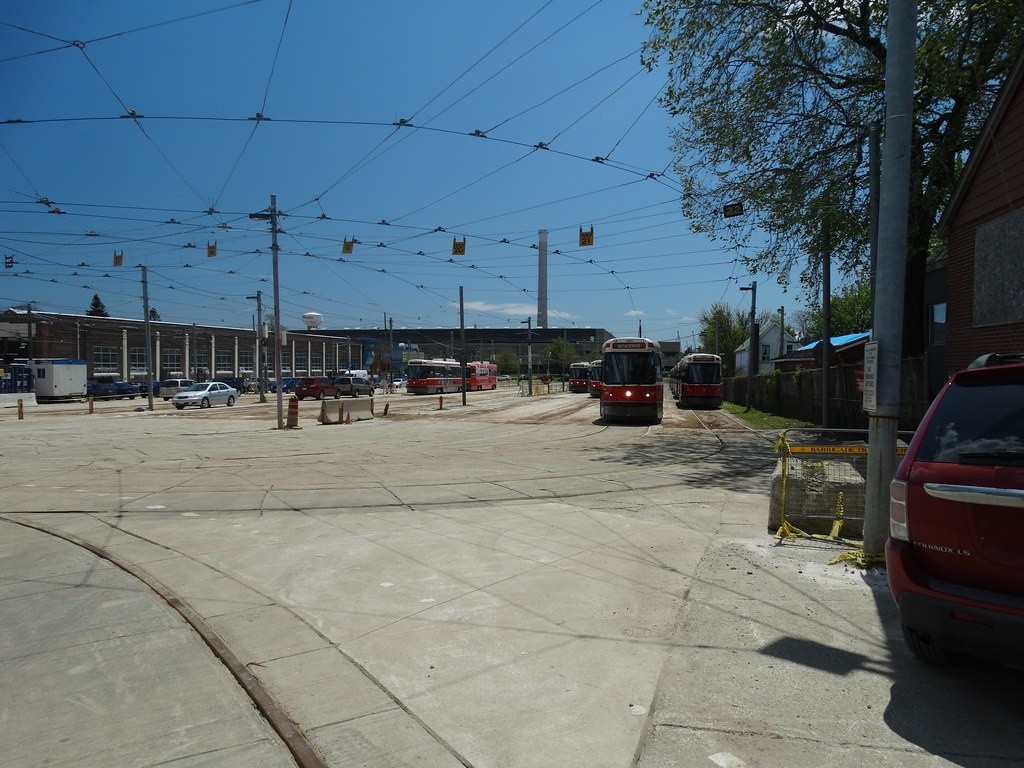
[213,377,244,396]
[344,369,368,380]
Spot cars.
[271,377,303,393]
[172,381,238,409]
[140,382,162,398]
[334,377,375,397]
[246,377,269,392]
[296,377,341,399]
[375,373,407,388]
[88,382,138,400]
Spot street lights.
[249,213,282,396]
[740,280,757,412]
[246,291,266,404]
[777,306,785,356]
[521,318,532,396]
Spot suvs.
[886,353,1017,653]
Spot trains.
[669,353,724,409]
[588,360,602,397]
[570,361,591,393]
[600,336,665,423]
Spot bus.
[406,358,466,394]
[467,361,497,391]
